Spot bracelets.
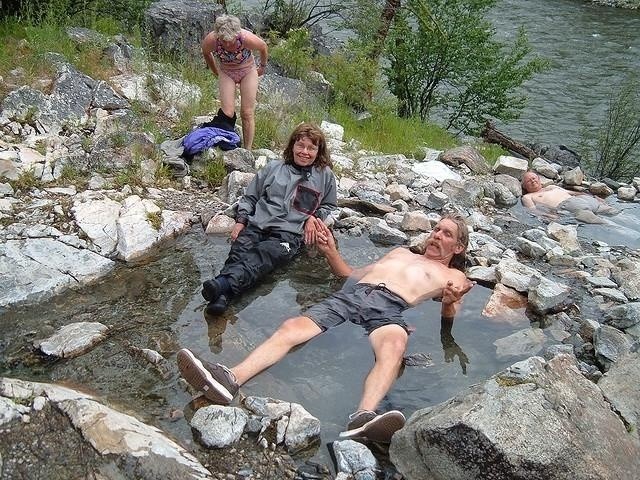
[259,63,267,67]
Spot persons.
[201,15,269,153]
[200,122,337,316]
[176,211,477,442]
[520,170,640,241]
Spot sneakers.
[177,349,239,404]
[202,280,220,301]
[205,299,226,315]
[338,410,406,443]
